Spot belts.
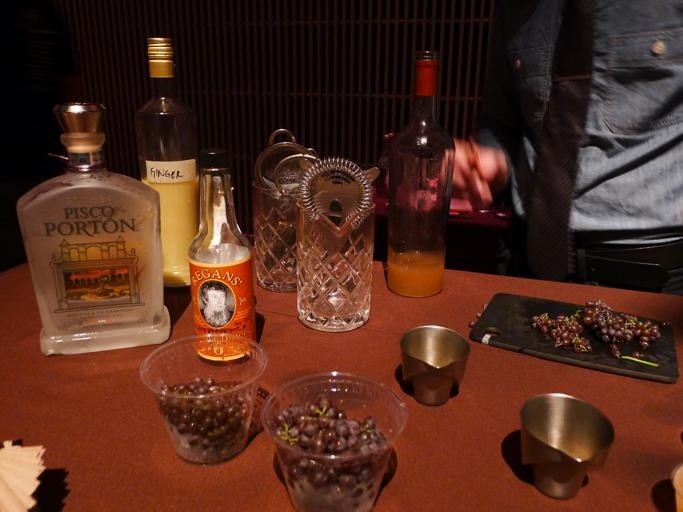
[567,242,683,287]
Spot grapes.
[531,299,672,360]
[159,377,250,460]
[274,396,385,506]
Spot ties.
[521,1,597,282]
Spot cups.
[519,392,615,500]
[251,130,376,335]
[260,368,409,510]
[401,325,472,406]
[141,336,270,465]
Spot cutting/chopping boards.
[469,291,678,389]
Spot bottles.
[386,49,455,299]
[135,36,198,286]
[19,95,170,356]
[185,146,255,362]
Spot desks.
[0,246,683,512]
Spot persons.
[199,282,232,327]
[436,0,682,294]
